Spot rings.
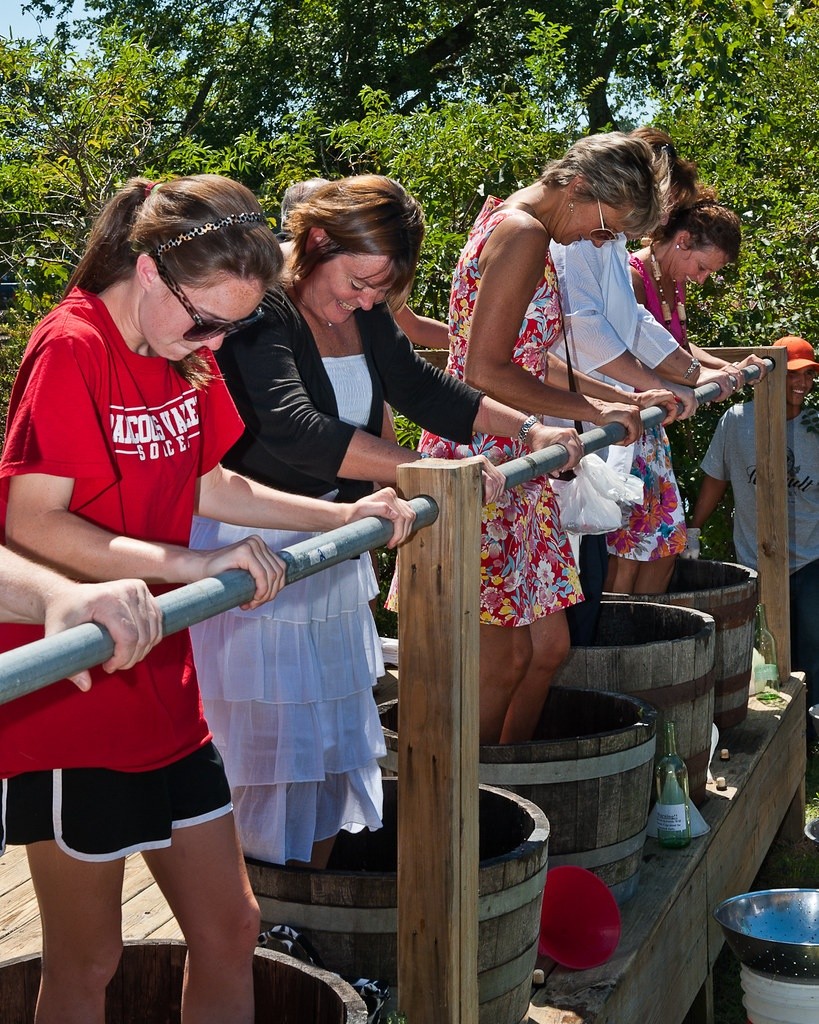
[732,386,736,391]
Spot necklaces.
[291,280,334,328]
[648,241,687,340]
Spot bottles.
[752,604,778,698]
[654,723,691,848]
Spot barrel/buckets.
[740,962,819,1024]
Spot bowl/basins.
[538,865,620,968]
[808,703,819,737]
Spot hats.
[771,335,819,373]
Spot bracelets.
[517,414,538,442]
[732,361,740,367]
[682,358,700,380]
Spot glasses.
[588,184,620,242]
[133,253,265,342]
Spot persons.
[0,174,418,1024]
[185,175,583,862]
[420,131,738,744]
[265,177,449,489]
[675,334,819,673]
[602,188,743,593]
